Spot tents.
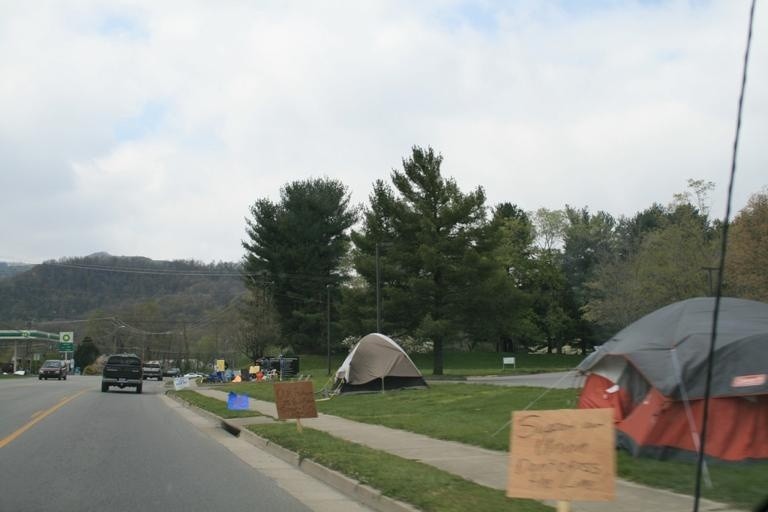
[572,295,768,469]
[323,331,431,398]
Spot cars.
[38,359,67,380]
[142,361,180,381]
[184,372,210,378]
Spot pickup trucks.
[102,355,142,393]
